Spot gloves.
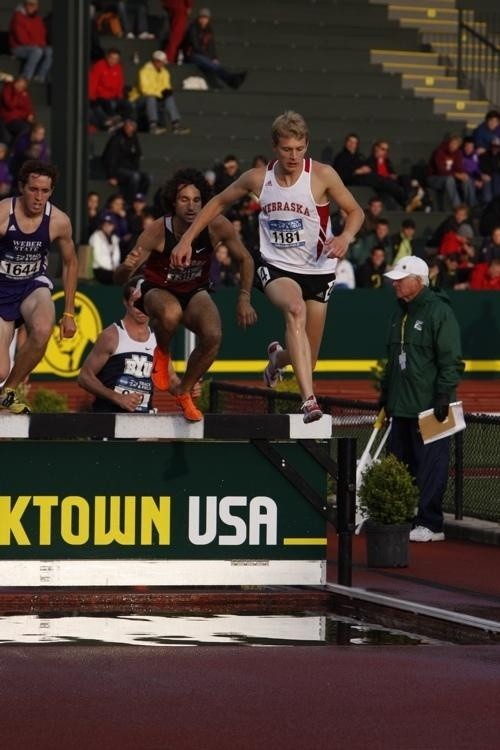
[432,394,451,423]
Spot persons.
[170,110,364,424]
[123,169,257,424]
[433,208,475,250]
[179,8,248,87]
[373,256,466,543]
[1,142,18,199]
[104,195,133,248]
[369,144,423,210]
[470,259,500,290]
[430,254,467,289]
[253,157,266,168]
[480,227,500,260]
[15,3,54,82]
[85,50,130,130]
[23,127,55,165]
[118,0,158,41]
[2,164,77,415]
[216,157,245,184]
[335,134,370,185]
[354,220,396,261]
[211,245,231,283]
[89,215,123,283]
[128,193,151,219]
[472,112,500,183]
[430,136,471,206]
[456,140,490,202]
[357,245,390,288]
[359,198,391,235]
[132,49,189,134]
[230,220,242,235]
[84,191,101,221]
[102,119,151,197]
[77,274,202,415]
[389,219,420,268]
[2,76,34,149]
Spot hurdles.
[1,407,357,585]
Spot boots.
[221,67,246,87]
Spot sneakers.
[173,125,193,135]
[140,32,148,39]
[150,345,171,391]
[405,195,421,213]
[150,124,166,134]
[300,394,323,423]
[409,524,446,543]
[126,32,134,39]
[175,393,201,421]
[0,387,32,413]
[263,341,284,389]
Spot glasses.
[376,144,389,152]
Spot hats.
[133,192,147,202]
[153,50,169,64]
[197,8,212,17]
[382,255,429,281]
[98,211,118,223]
